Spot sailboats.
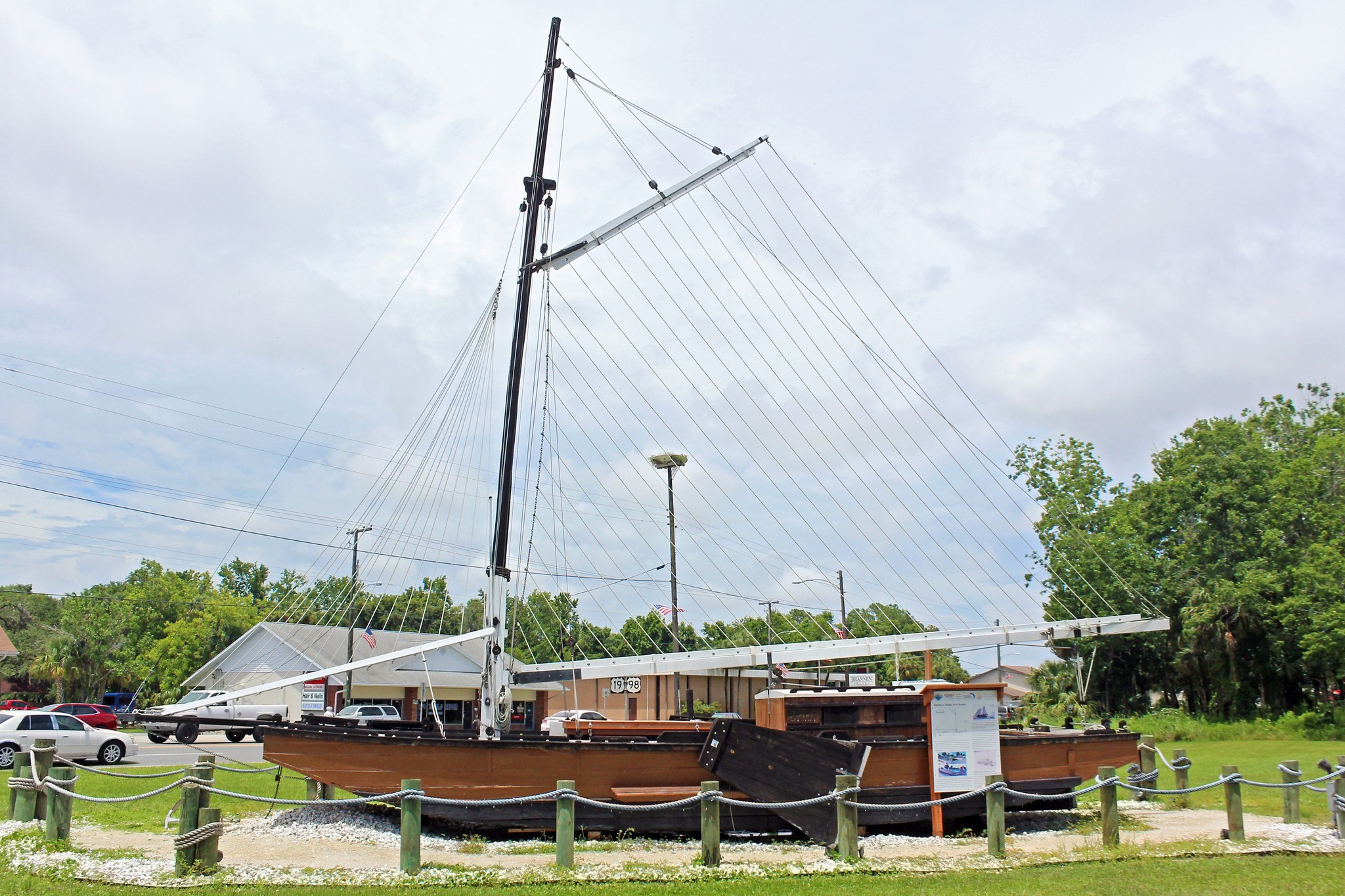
[130,0,1175,837]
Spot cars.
[1,699,38,714]
[0,710,137,768]
[712,713,743,721]
[540,709,611,735]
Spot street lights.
[794,569,850,639]
[347,579,381,701]
[650,453,687,650]
[759,598,797,648]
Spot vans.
[92,693,138,718]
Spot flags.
[360,628,377,650]
[653,604,688,616]
[827,621,850,639]
[774,663,790,678]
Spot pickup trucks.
[145,687,289,746]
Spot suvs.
[998,704,1018,723]
[329,700,400,727]
[31,703,118,733]
